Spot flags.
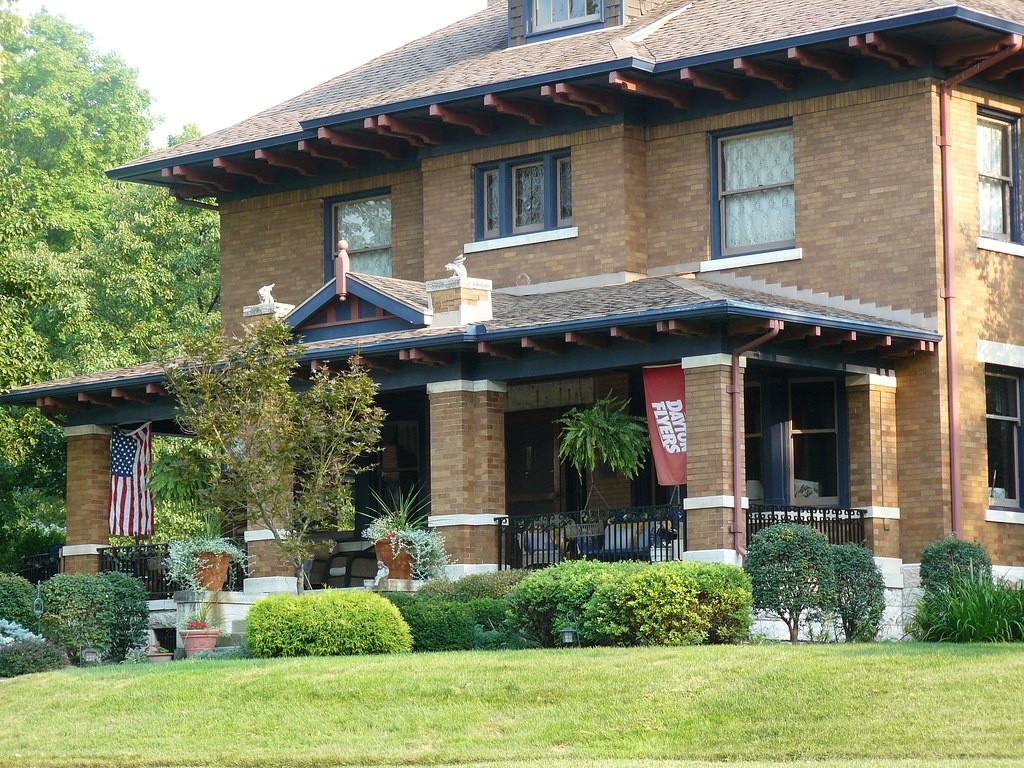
[108,422,155,536]
[642,364,688,486]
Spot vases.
[577,535,603,553]
[147,653,174,663]
[180,630,218,659]
[584,517,597,523]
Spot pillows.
[527,532,554,550]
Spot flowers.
[145,642,169,654]
[583,510,597,517]
[166,592,237,645]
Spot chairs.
[514,515,578,568]
[320,528,376,588]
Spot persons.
[374,561,390,587]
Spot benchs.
[601,513,672,561]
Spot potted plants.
[623,507,638,521]
[654,508,668,520]
[356,479,458,580]
[155,538,245,591]
[651,531,673,565]
[640,510,652,520]
[607,508,621,524]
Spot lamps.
[557,612,581,647]
[80,636,95,669]
